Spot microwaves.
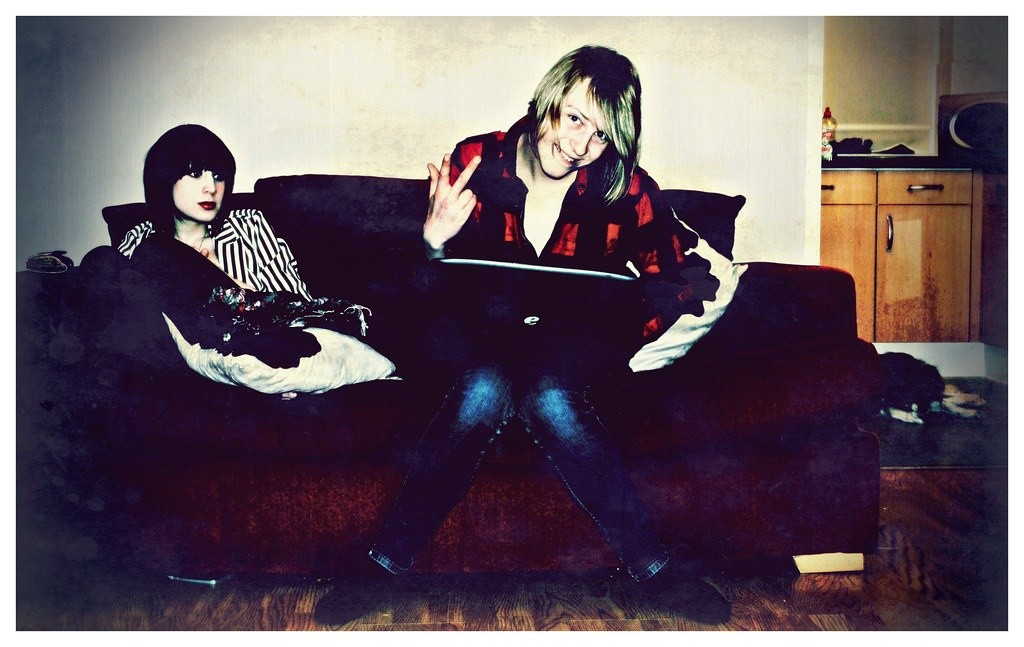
[937,91,1008,158]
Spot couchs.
[15,175,878,613]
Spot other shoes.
[308,581,389,625]
[628,570,732,628]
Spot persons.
[306,46,802,625]
[105,123,406,412]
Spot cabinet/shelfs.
[877,171,971,344]
[821,169,874,345]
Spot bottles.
[821,107,838,163]
